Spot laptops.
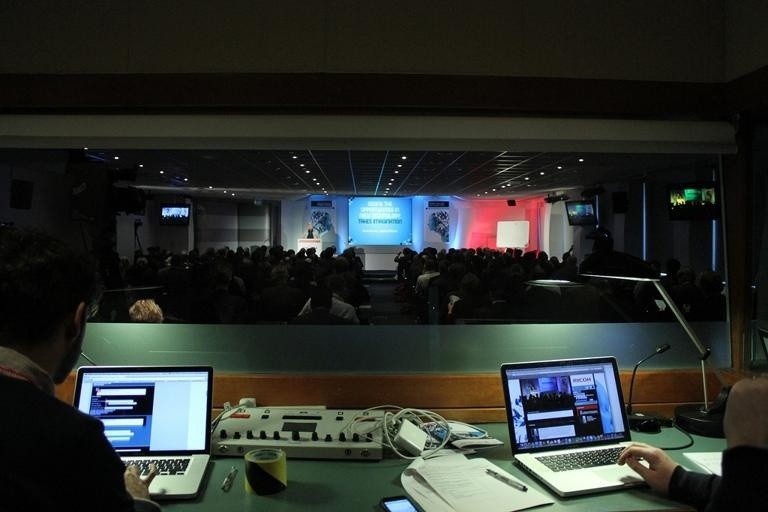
[500,356,689,498]
[72,364,214,500]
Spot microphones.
[624,343,673,427]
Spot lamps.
[526,266,634,323]
[577,249,726,438]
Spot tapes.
[244,448,287,497]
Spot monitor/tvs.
[666,180,720,222]
[565,199,599,226]
[157,202,191,225]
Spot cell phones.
[380,494,420,511]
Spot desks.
[117,415,726,512]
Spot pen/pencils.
[486,468,527,491]
[220,465,238,490]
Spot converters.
[392,417,429,456]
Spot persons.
[392,248,578,322]
[118,241,362,326]
[0,248,162,512]
[617,377,767,512]
[305,222,316,238]
